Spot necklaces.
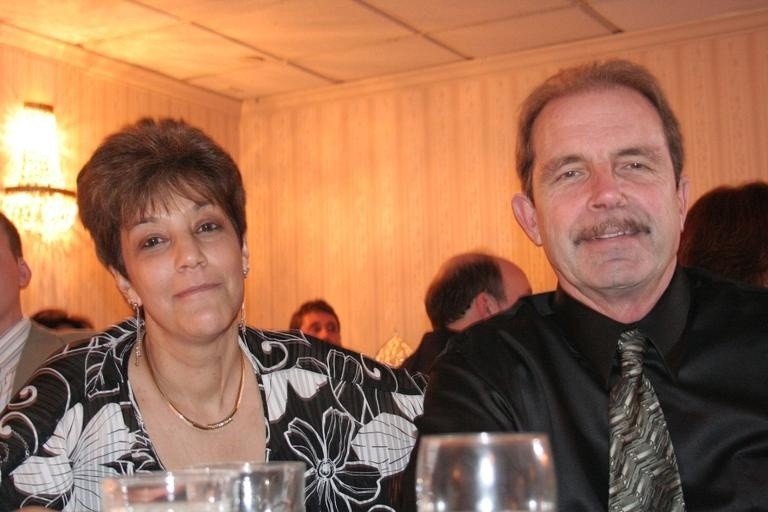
[139,330,248,431]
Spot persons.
[675,179,767,290]
[0,214,95,420]
[397,252,534,375]
[1,114,431,510]
[397,59,767,511]
[288,300,342,348]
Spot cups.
[413,428,560,512]
[98,460,308,512]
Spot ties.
[609,331,685,510]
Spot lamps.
[4,102,76,198]
